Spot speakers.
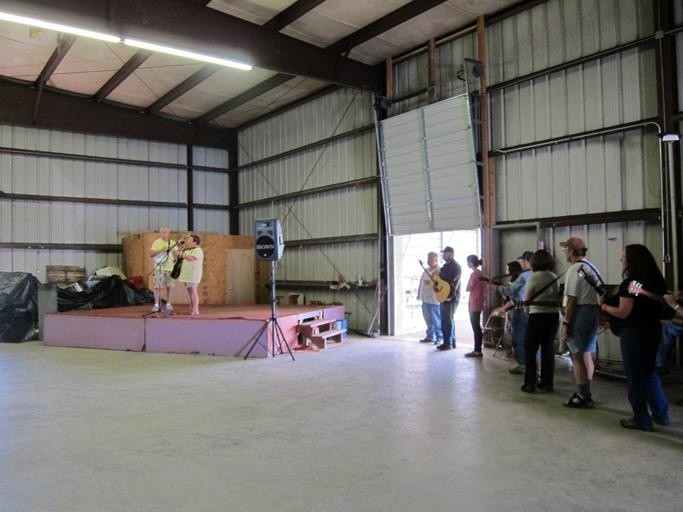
[254,218,285,261]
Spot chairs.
[479,306,509,358]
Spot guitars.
[510,298,610,329]
[629,280,682,311]
[478,274,505,286]
[418,259,455,303]
[154,235,191,263]
[170,247,185,278]
[578,265,621,338]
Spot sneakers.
[166,305,172,313]
[521,384,535,393]
[509,366,526,374]
[537,382,552,391]
[620,417,651,431]
[152,306,160,312]
[649,414,669,426]
[453,343,455,348]
[420,338,435,342]
[562,392,592,408]
[437,344,452,350]
[435,339,443,345]
[465,351,483,357]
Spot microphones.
[166,240,185,254]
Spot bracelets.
[673,304,679,311]
[600,303,608,312]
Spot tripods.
[244,263,297,362]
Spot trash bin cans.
[335,320,348,339]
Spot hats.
[517,252,533,260]
[560,237,587,255]
[440,247,454,252]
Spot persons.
[463,254,485,359]
[520,250,561,393]
[496,262,521,360]
[178,235,206,313]
[436,246,462,351]
[149,227,179,313]
[417,252,444,344]
[559,237,600,410]
[663,294,683,317]
[597,244,675,431]
[490,250,533,374]
